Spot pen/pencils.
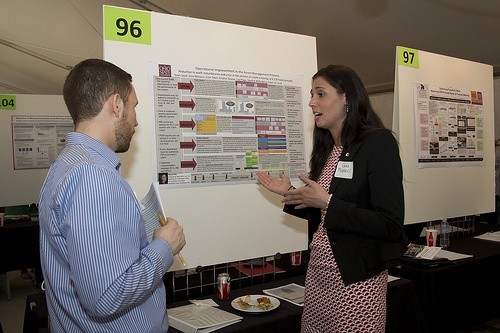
[158,214,186,266]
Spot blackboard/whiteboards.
[102,4,318,272]
[0,94,74,207]
[392,46,495,225]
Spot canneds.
[217,273,230,300]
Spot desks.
[167,274,412,333]
[400,237,500,333]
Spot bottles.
[439,217,450,247]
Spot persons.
[38,59,186,333]
[256,65,409,333]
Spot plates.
[231,295,280,313]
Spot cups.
[426,230,438,247]
[0,213,5,227]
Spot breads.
[257,297,270,304]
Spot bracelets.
[325,194,333,209]
[284,186,293,197]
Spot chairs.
[0,217,44,300]
